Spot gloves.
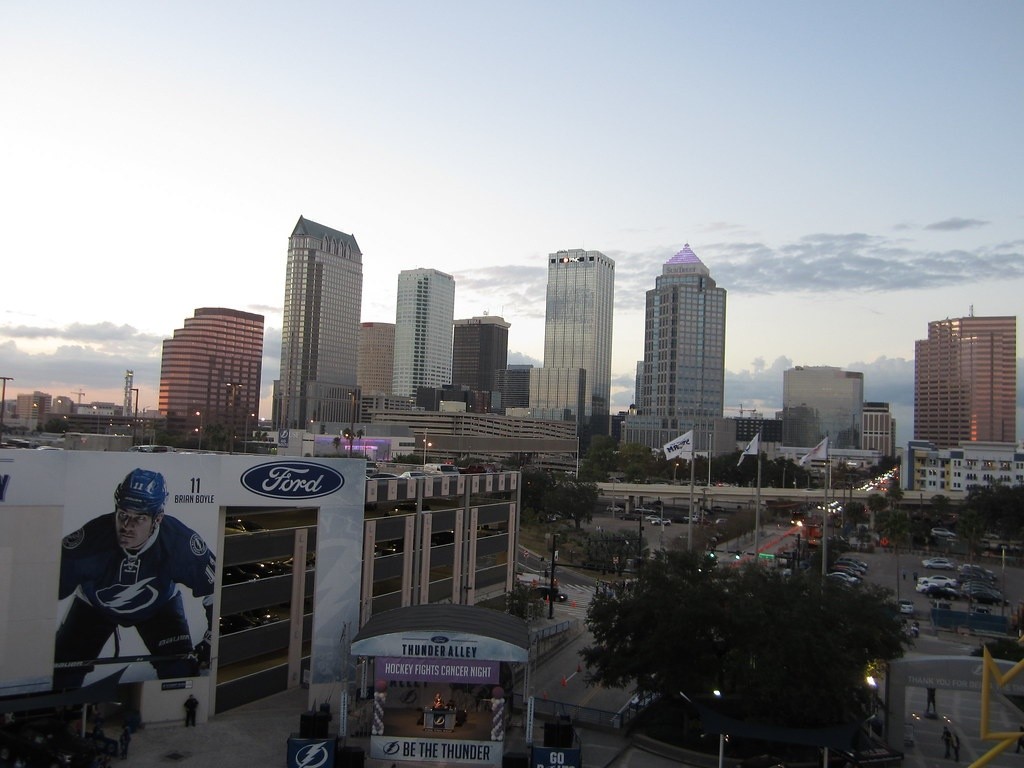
[185,640,210,669]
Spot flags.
[799,436,829,467]
[736,433,759,467]
[663,429,693,461]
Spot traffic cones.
[561,676,568,686]
[546,595,549,603]
[572,601,576,608]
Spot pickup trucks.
[0,719,96,768]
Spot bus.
[128,445,177,452]
[424,463,460,475]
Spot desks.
[423,712,457,731]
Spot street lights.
[790,514,804,567]
[548,532,560,620]
[226,382,243,456]
[244,413,255,453]
[609,477,620,518]
[348,391,355,458]
[0,377,13,425]
[129,386,138,446]
[674,463,679,485]
[829,441,833,491]
[708,434,711,487]
[867,676,877,738]
[423,432,432,471]
[817,501,843,537]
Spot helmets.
[114,468,169,511]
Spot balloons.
[371,680,388,735]
[491,687,505,741]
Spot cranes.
[71,389,85,403]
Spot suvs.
[651,518,671,526]
[716,519,728,523]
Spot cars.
[826,527,1010,613]
[607,505,661,521]
[222,552,315,585]
[530,588,568,602]
[538,511,576,524]
[684,515,712,525]
[219,609,281,634]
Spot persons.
[941,726,951,759]
[912,619,919,637]
[119,724,132,760]
[912,569,918,582]
[432,692,443,707]
[75,712,105,735]
[184,694,199,728]
[52,468,217,691]
[926,687,936,712]
[901,568,908,581]
[1014,726,1024,753]
[951,732,960,762]
